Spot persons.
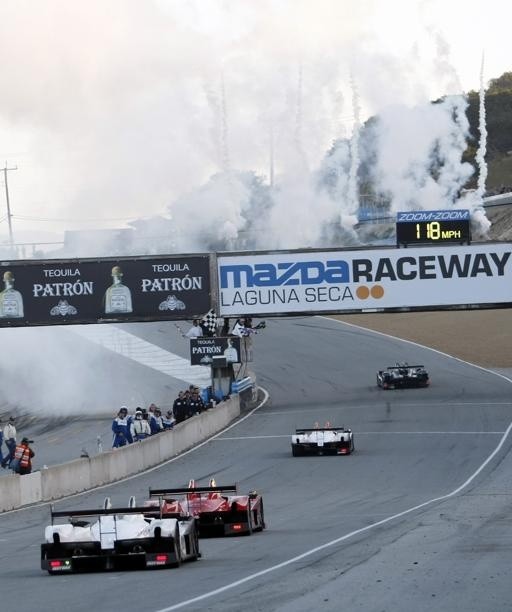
[183,318,203,337]
[112,382,216,451]
[230,318,244,337]
[0,422,9,469]
[0,415,17,469]
[13,436,35,476]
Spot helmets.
[120,406,129,414]
[135,411,142,415]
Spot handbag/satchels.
[10,459,21,473]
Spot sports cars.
[376,361,430,390]
[145,478,265,537]
[291,421,355,455]
[39,495,200,574]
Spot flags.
[238,326,260,338]
[202,308,220,339]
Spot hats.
[21,437,34,443]
